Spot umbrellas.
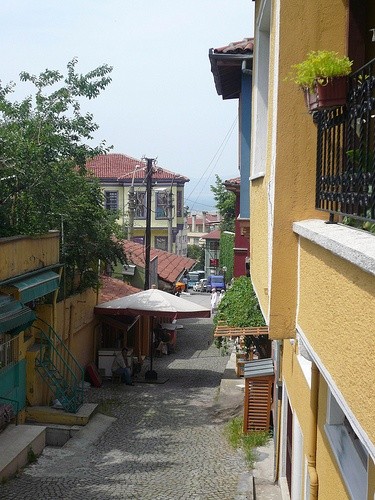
[94,284,211,370]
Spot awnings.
[11,268,61,303]
[0,293,36,336]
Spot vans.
[200,278,208,286]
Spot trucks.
[204,275,225,295]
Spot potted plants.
[282,48,353,111]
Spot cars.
[192,282,207,293]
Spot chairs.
[111,369,122,384]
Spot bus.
[188,271,205,288]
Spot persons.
[153,324,178,355]
[182,274,190,292]
[209,287,225,318]
[112,347,136,386]
